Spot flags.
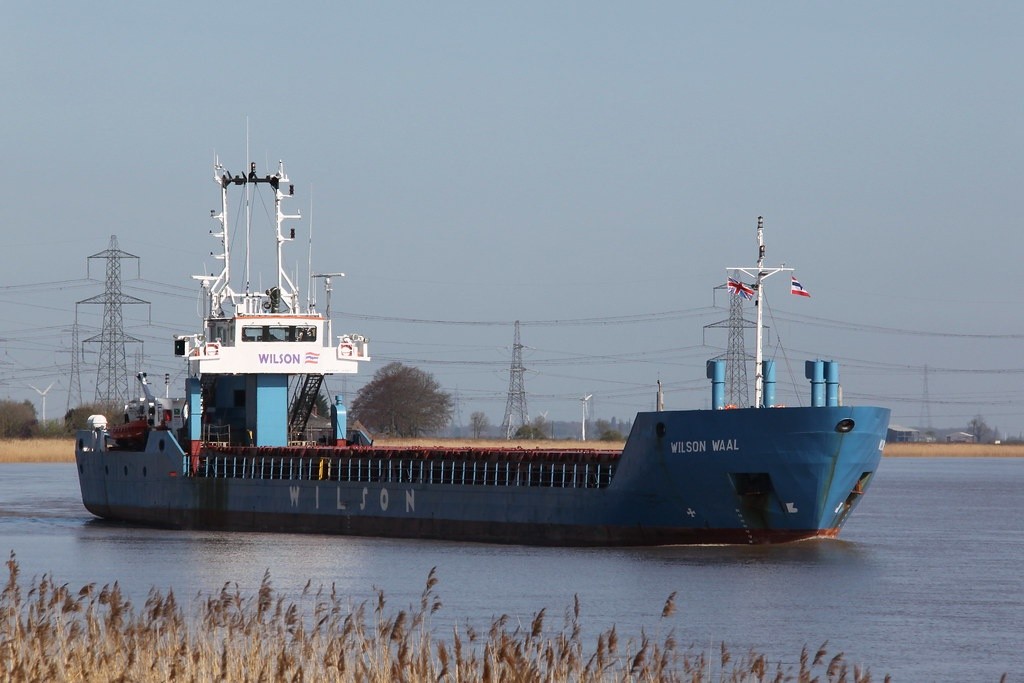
[791,276,810,297]
[728,276,754,301]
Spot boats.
[70,115,894,553]
